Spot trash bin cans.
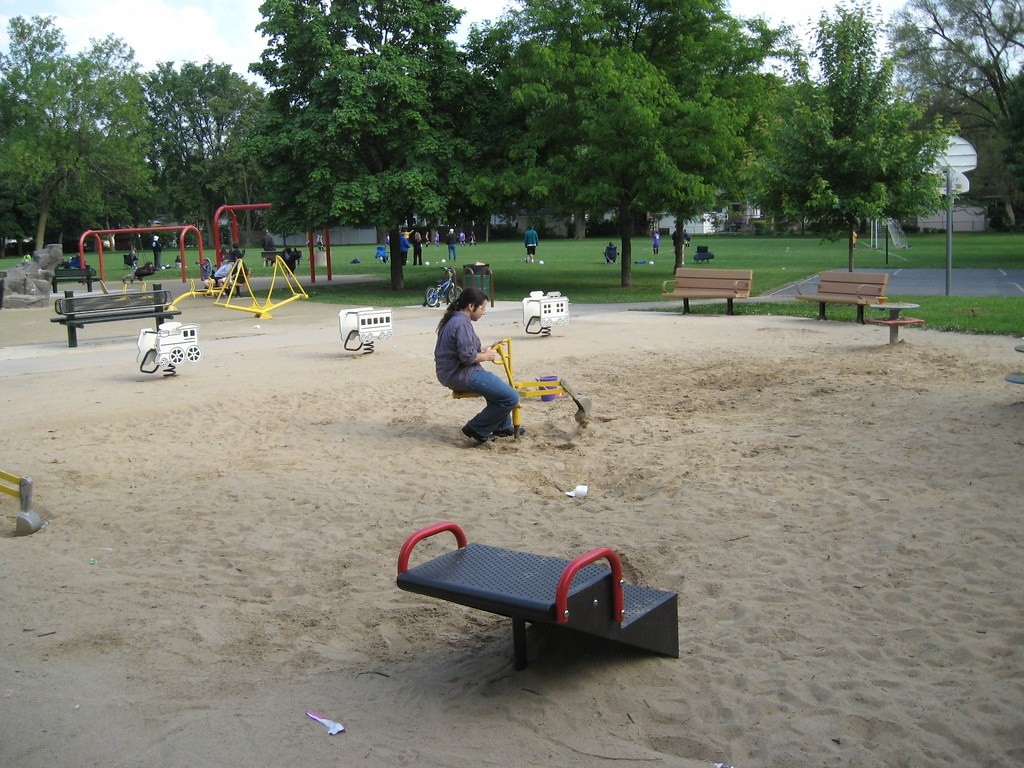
[463,263,490,300]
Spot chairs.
[232,267,250,298]
[694,245,709,264]
[603,246,619,265]
[123,254,132,270]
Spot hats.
[220,254,231,259]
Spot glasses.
[479,305,486,310]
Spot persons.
[124,249,139,269]
[413,232,423,265]
[387,227,414,266]
[262,230,276,267]
[22,252,31,261]
[524,225,538,263]
[282,247,301,276]
[152,236,162,271]
[446,229,457,262]
[684,229,692,247]
[425,230,477,248]
[603,242,620,264]
[122,262,156,283]
[434,287,525,444]
[317,234,325,252]
[175,255,187,268]
[203,243,248,299]
[853,229,858,250]
[652,230,660,255]
[672,225,685,266]
[70,253,81,268]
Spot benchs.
[661,268,753,315]
[260,251,302,266]
[50,283,181,348]
[795,271,889,324]
[51,268,102,293]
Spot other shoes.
[492,427,525,437]
[222,294,227,298]
[462,424,495,442]
